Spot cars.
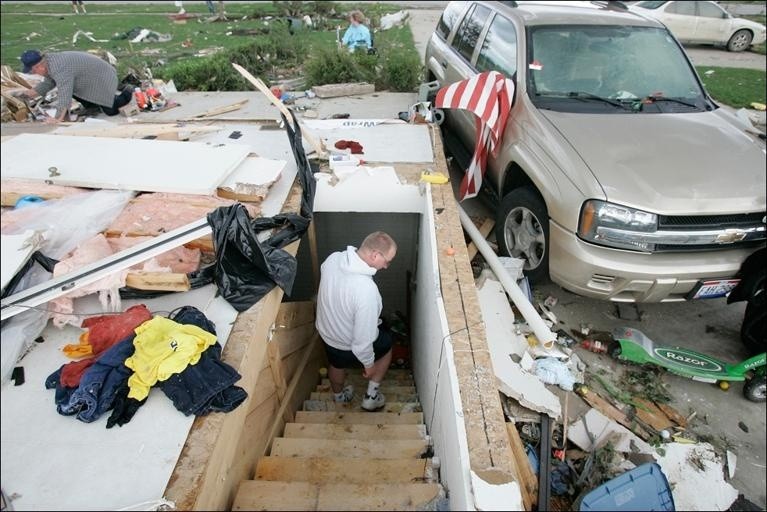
[627,0,766,53]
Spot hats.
[21,49,43,73]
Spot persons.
[337,9,373,56]
[14,49,138,126]
[312,230,401,414]
[70,1,88,16]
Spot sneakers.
[132,85,152,110]
[335,385,353,402]
[362,393,386,409]
[70,106,100,116]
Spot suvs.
[422,1,766,302]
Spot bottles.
[582,340,608,355]
[328,156,367,170]
[421,170,454,184]
[134,87,146,109]
